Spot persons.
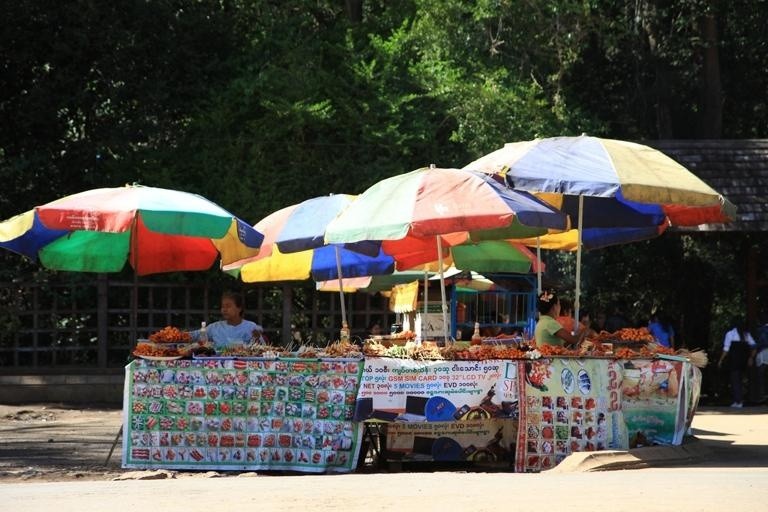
[717,315,758,408]
[648,311,674,347]
[497,313,519,336]
[557,300,612,347]
[185,293,268,345]
[535,293,580,349]
[371,321,381,335]
[747,311,768,406]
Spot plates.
[424,396,458,421]
[139,353,183,361]
[576,368,591,396]
[560,367,575,395]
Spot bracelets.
[749,354,754,359]
[719,358,724,361]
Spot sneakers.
[730,400,744,408]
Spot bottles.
[471,321,482,344]
[340,324,351,343]
[199,321,207,346]
